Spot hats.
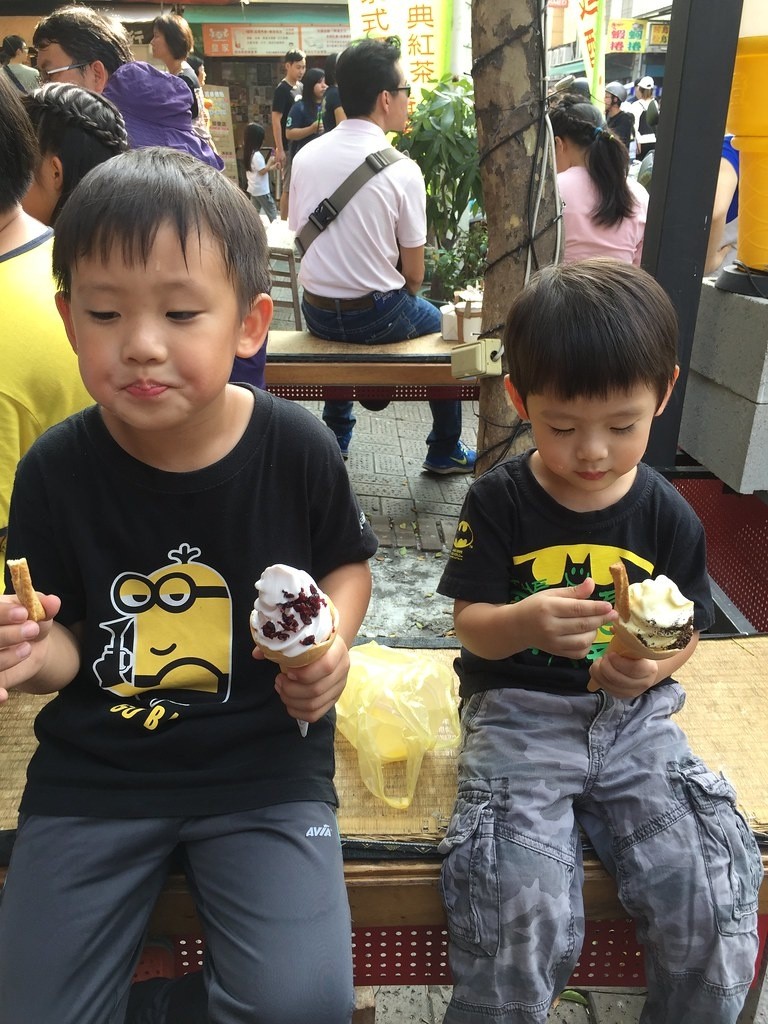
[638,76,654,89]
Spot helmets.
[569,76,590,100]
[605,81,628,102]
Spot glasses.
[380,83,411,97]
[39,60,93,86]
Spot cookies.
[6,556,46,621]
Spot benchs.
[260,330,481,403]
[1,634,768,1024]
[265,218,308,330]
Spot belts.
[303,288,374,310]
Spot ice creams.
[587,562,695,693]
[250,564,340,737]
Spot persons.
[288,40,476,474]
[242,122,280,222]
[546,74,739,276]
[0,146,378,1024]
[435,257,764,1024]
[0,5,265,597]
[271,47,347,220]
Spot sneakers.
[335,435,350,458]
[421,439,477,474]
